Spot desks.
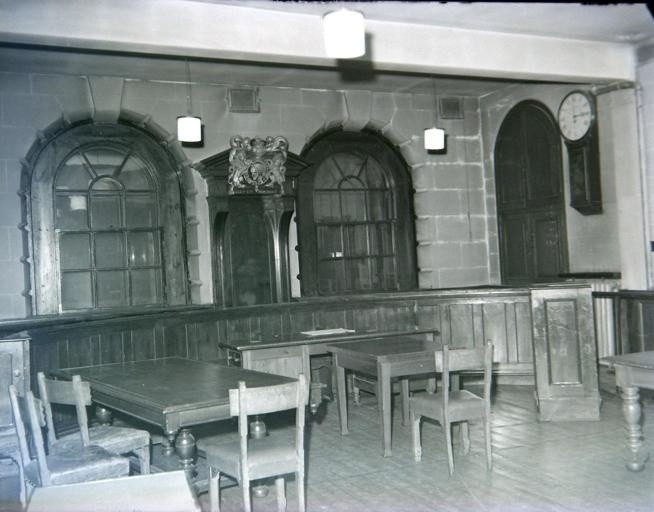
[48,355,301,452]
[219,324,419,428]
[597,350,654,471]
[26,468,203,512]
[324,335,471,458]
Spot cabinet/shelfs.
[0,330,31,468]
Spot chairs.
[192,373,308,512]
[7,384,130,487]
[405,338,494,476]
[35,370,153,475]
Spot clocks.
[556,89,603,215]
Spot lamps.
[70,197,88,211]
[423,80,445,150]
[176,57,201,143]
[322,2,367,58]
